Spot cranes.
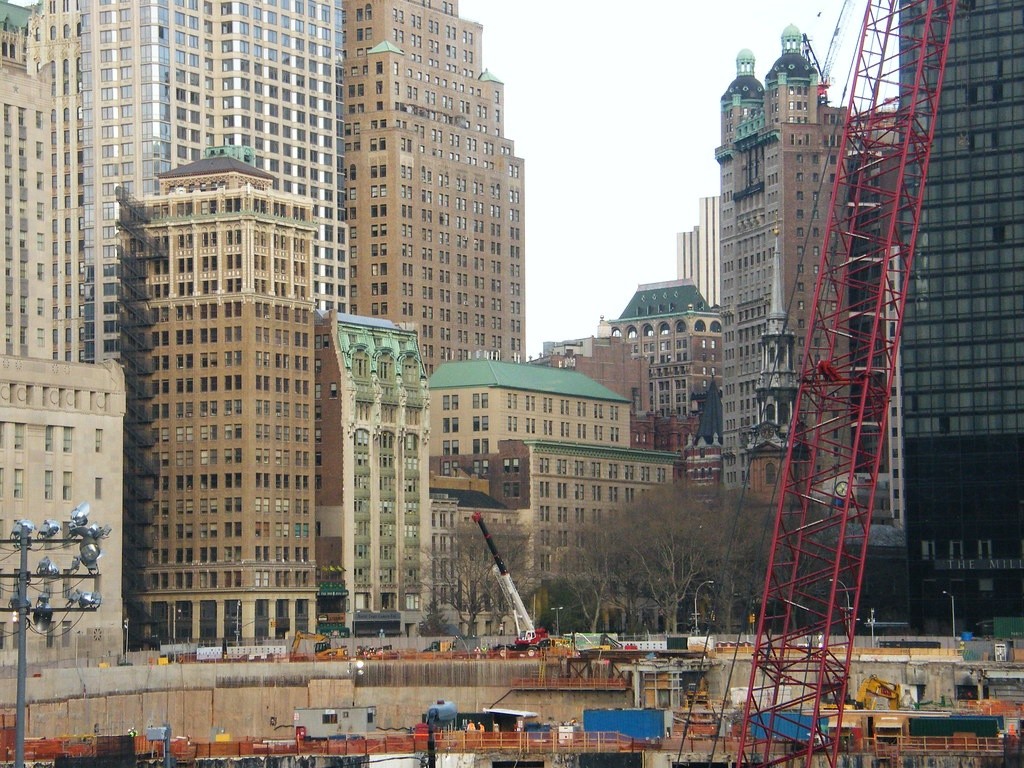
[820,0,853,89]
[736,0,965,768]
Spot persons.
[475,647,481,660]
[477,722,485,731]
[467,720,475,731]
[131,727,138,737]
[481,646,488,659]
[128,729,134,737]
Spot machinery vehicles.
[683,675,712,710]
[471,511,550,651]
[822,673,901,712]
[290,630,348,658]
[424,641,452,652]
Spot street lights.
[695,581,714,636]
[235,599,241,647]
[551,607,563,636]
[944,591,956,652]
[124,618,129,662]
[829,578,851,632]
